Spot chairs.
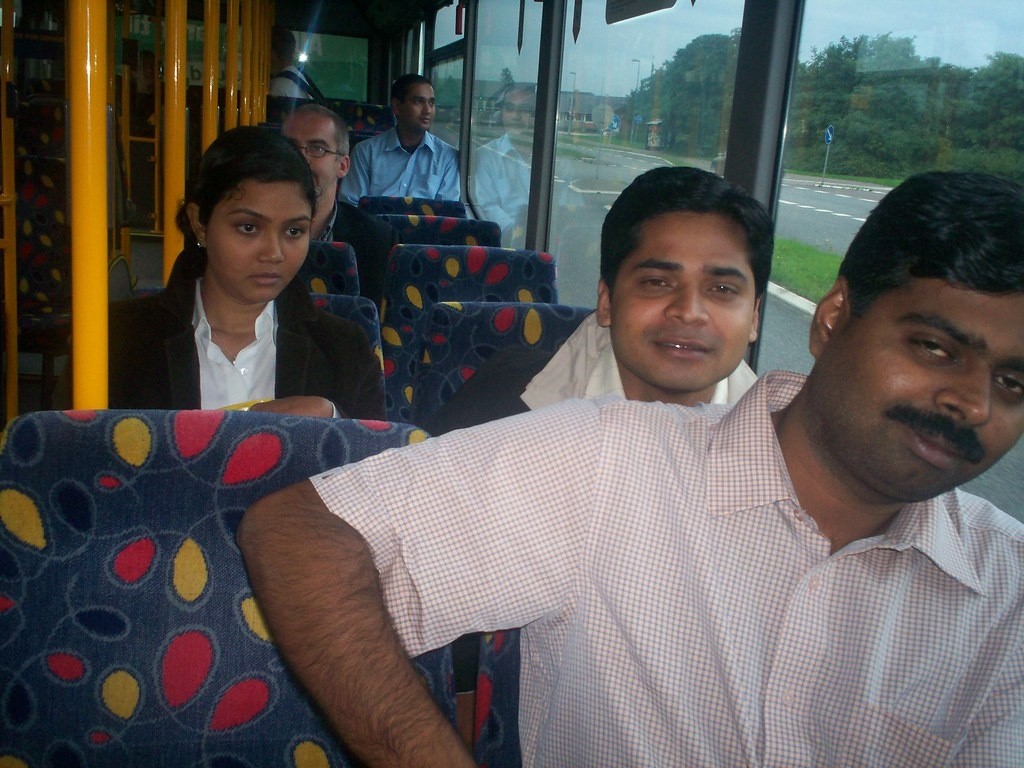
[0,83,600,768]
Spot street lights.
[566,71,577,136]
[630,58,640,146]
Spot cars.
[710,149,785,182]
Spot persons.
[238,169,1024,768]
[468,83,583,252]
[457,165,774,428]
[114,30,463,421]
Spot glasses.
[288,139,345,160]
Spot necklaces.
[210,325,237,365]
